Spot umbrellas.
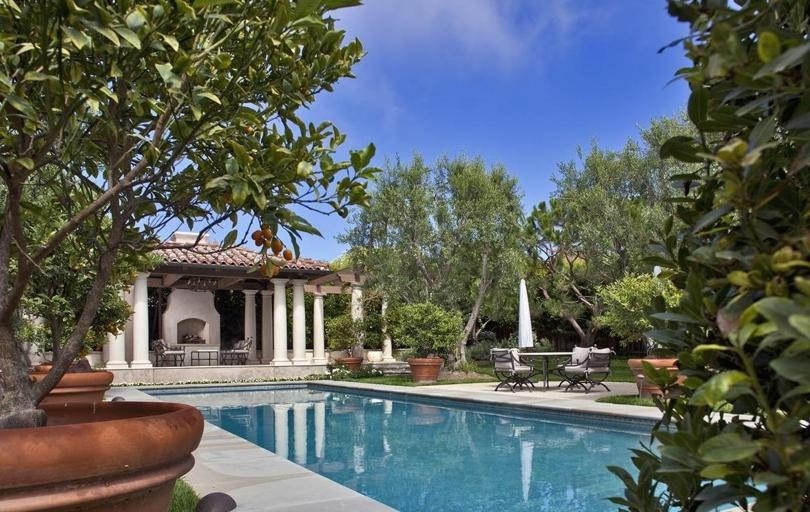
[517,277,534,349]
[517,437,536,504]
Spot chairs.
[489,347,615,394]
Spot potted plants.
[0,0,465,512]
[591,272,691,400]
